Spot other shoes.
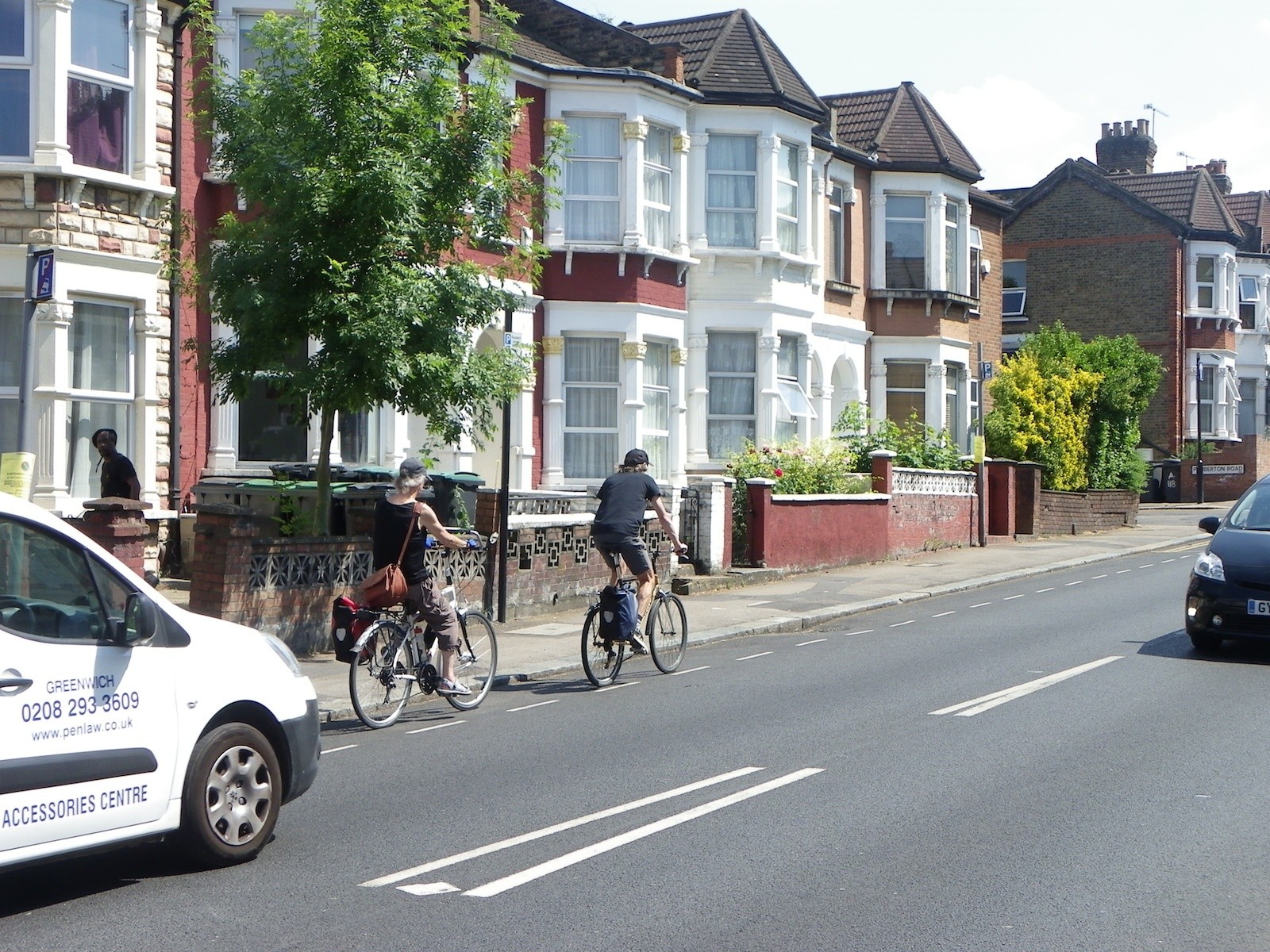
[634,629,644,641]
[629,629,649,655]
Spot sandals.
[437,677,472,696]
[380,647,407,675]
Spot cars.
[1183,472,1269,650]
[0,489,323,874]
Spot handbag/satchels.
[360,564,407,610]
[599,585,638,641]
[331,595,381,666]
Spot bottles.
[414,626,427,662]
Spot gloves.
[467,538,476,549]
[425,537,434,547]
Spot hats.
[623,449,653,469]
[92,429,117,446]
[399,457,426,478]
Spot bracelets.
[464,540,469,550]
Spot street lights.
[1195,350,1222,503]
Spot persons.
[373,457,480,697]
[590,448,688,653]
[91,429,141,501]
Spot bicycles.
[581,547,689,688]
[349,546,499,731]
